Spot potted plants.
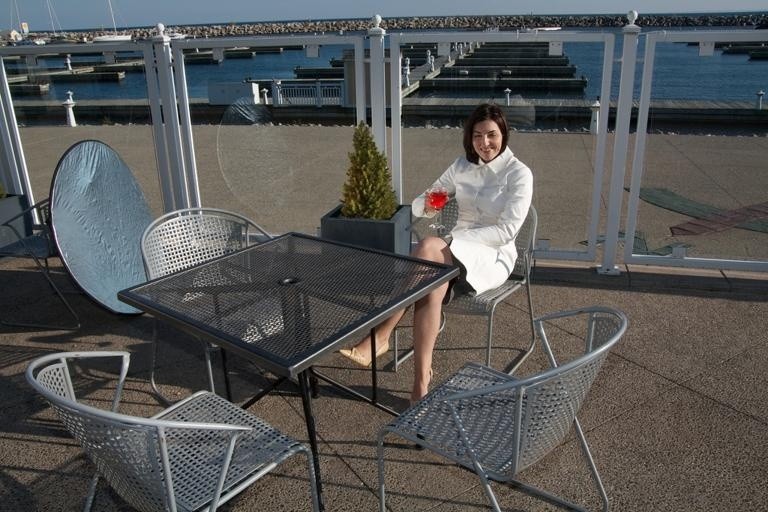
[318,117,416,259]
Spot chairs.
[379,298,631,512]
[11,350,323,510]
[391,193,544,378]
[139,206,297,388]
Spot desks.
[117,230,461,512]
[0,138,157,331]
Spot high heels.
[409,368,433,419]
[338,341,389,368]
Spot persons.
[336,102,535,421]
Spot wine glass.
[427,187,449,230]
